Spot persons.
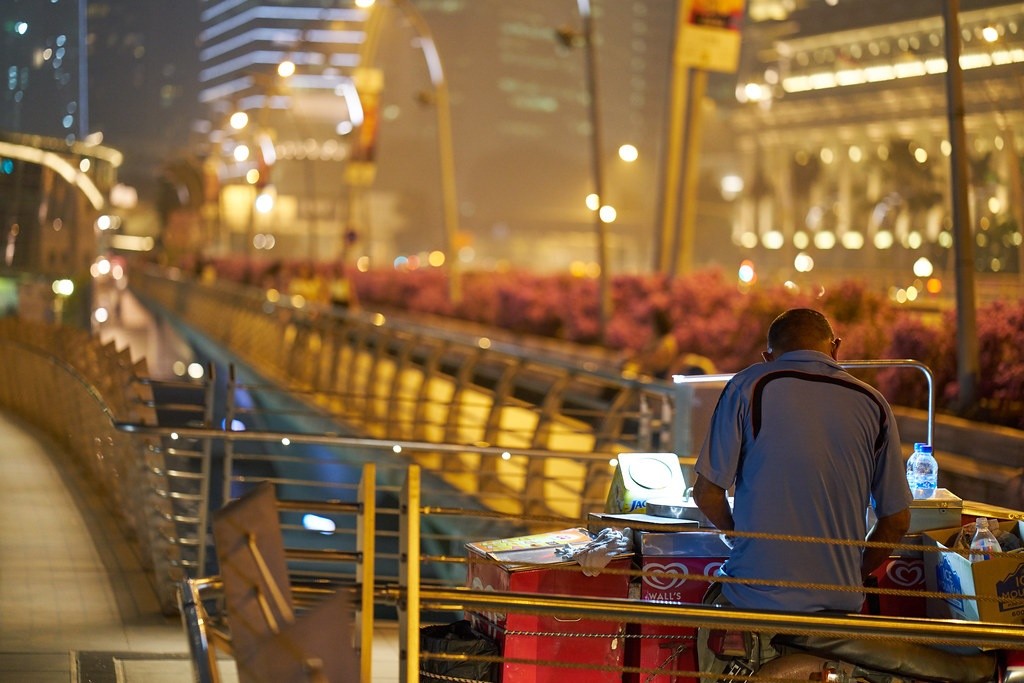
[691,307,913,682]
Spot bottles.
[968,517,1003,563]
[905,443,926,488]
[914,445,938,490]
